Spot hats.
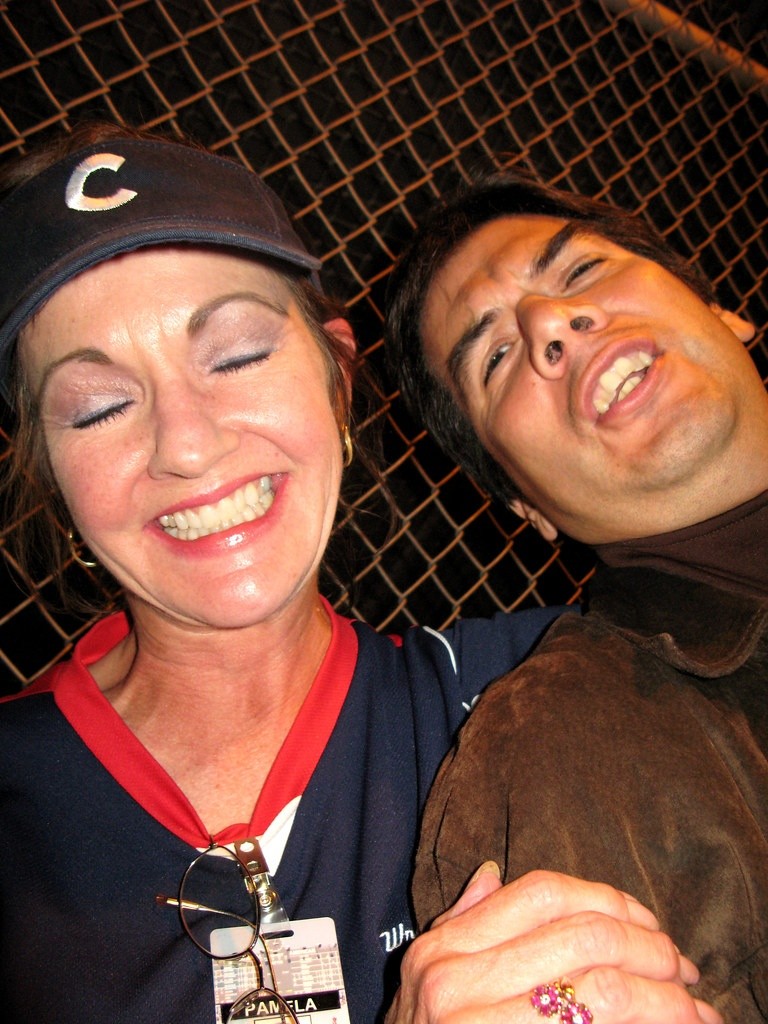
[0,136,323,343]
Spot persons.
[381,173,767,1024]
[0,119,576,1024]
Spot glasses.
[156,833,300,1024]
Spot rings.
[531,976,595,1023]
